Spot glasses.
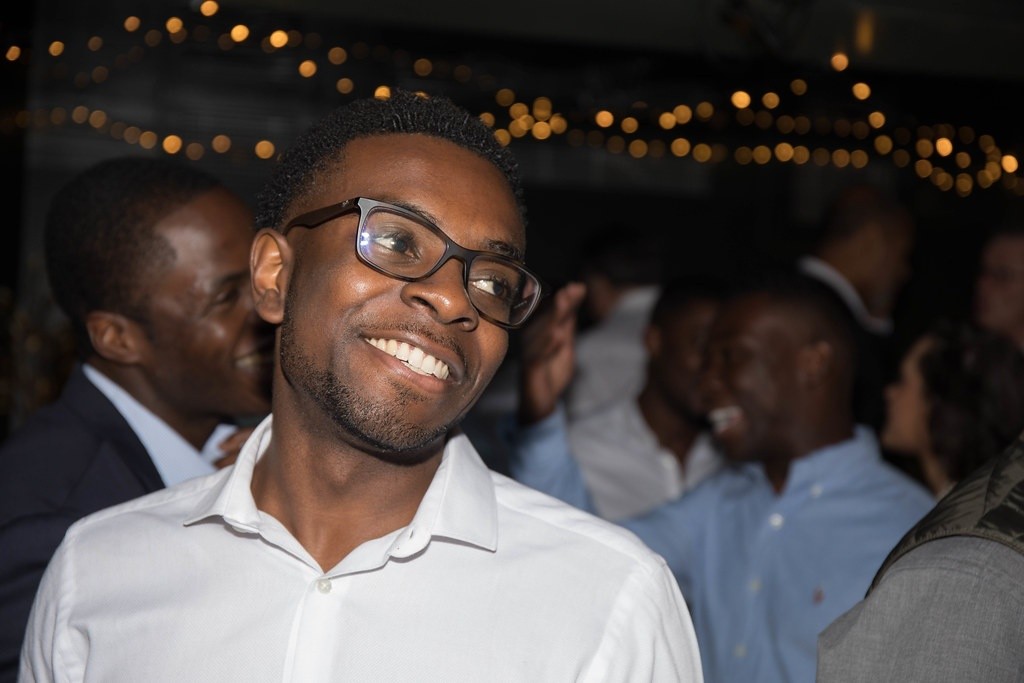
[283,196,542,329]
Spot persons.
[0,94,1024,683]
